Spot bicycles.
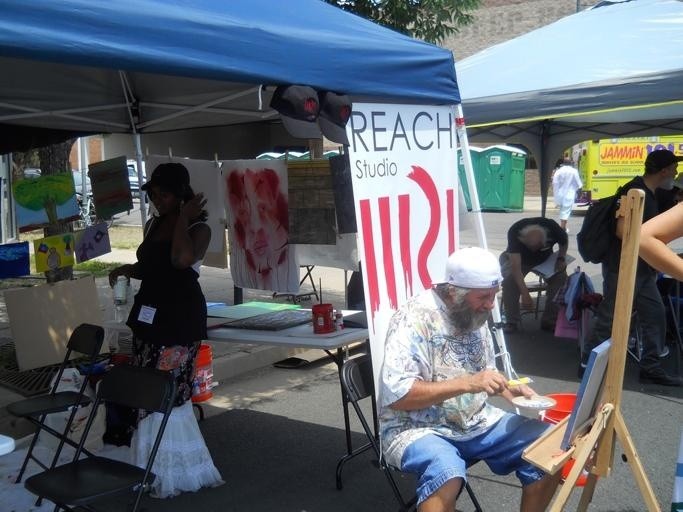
[73,190,114,229]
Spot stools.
[502,279,552,324]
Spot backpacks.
[576,180,639,265]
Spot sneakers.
[540,319,555,335]
[578,362,589,379]
[638,365,683,387]
[503,322,518,334]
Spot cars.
[2,166,92,205]
[125,165,148,204]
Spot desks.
[207,299,382,494]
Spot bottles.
[332,307,344,332]
[113,278,128,320]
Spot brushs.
[508,376,534,386]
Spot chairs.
[6,320,111,483]
[340,355,484,511]
[22,366,182,511]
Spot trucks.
[562,135,683,209]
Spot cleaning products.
[335,308,344,327]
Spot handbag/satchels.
[554,303,590,340]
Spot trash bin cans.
[457,145,528,212]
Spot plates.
[511,395,556,408]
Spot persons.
[374,242,565,512]
[549,156,586,230]
[106,162,211,502]
[223,166,287,276]
[635,199,683,286]
[496,213,567,334]
[572,148,683,387]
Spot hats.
[139,162,191,190]
[318,88,354,147]
[644,149,683,171]
[268,83,324,143]
[429,244,505,290]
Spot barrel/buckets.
[577,190,591,202]
[189,343,214,404]
[541,392,602,486]
